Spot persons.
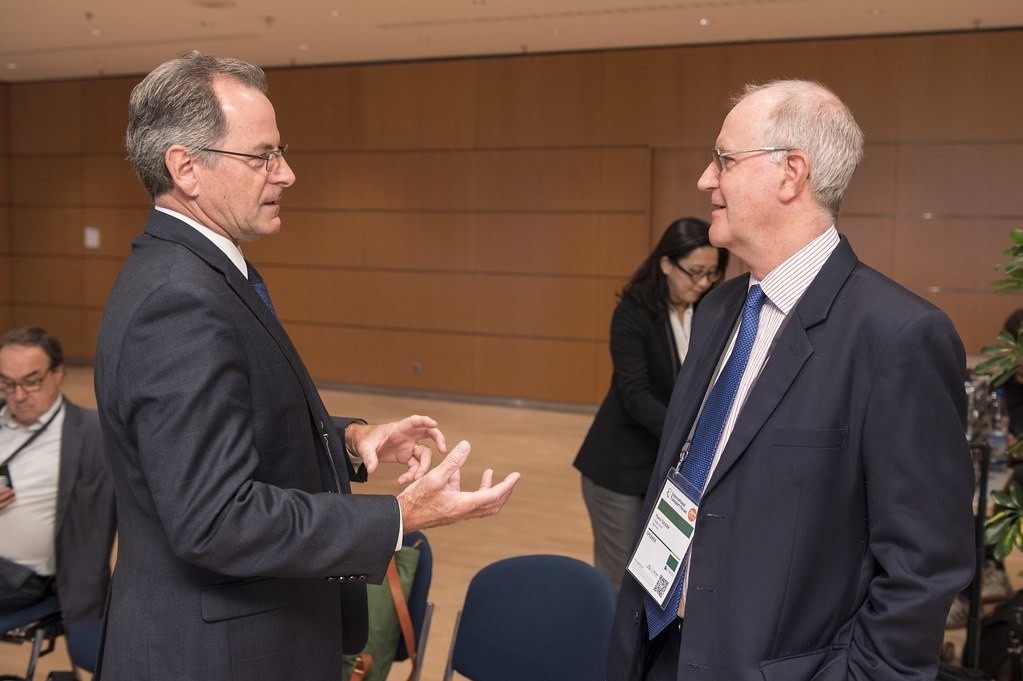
[0,325,120,675]
[603,78,978,681]
[572,216,731,595]
[943,307,1023,629]
[90,50,520,681]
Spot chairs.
[393,530,435,681]
[443,554,616,681]
[0,593,65,681]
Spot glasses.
[0,368,51,394]
[665,254,722,284]
[184,144,290,172]
[710,146,792,175]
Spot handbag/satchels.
[342,544,418,681]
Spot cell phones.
[0,464,17,496]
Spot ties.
[243,258,277,317]
[643,284,768,641]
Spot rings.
[413,442,420,453]
